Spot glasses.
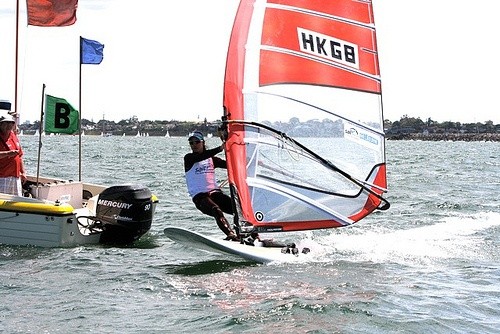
[189,141,202,145]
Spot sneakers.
[224,235,234,240]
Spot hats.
[0,114,15,123]
[188,131,203,140]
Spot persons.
[0,114,27,197]
[184,132,264,244]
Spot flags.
[45,94,80,135]
[25,0,80,27]
[82,37,105,64]
